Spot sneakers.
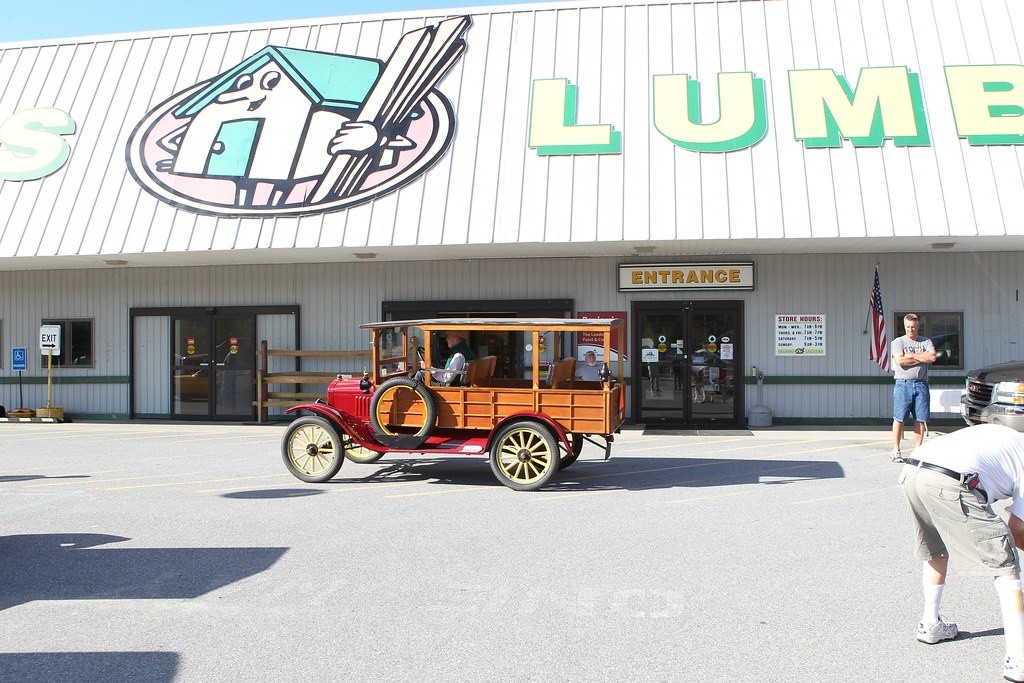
[916,614,958,644]
[1003,656,1024,682]
[890,449,903,463]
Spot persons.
[897,424,1024,683]
[574,350,602,381]
[670,352,681,391]
[220,347,238,409]
[646,347,663,393]
[414,330,478,387]
[890,314,937,464]
[694,355,725,404]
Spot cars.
[283,317,627,492]
[927,334,959,365]
[174,361,254,400]
[694,329,733,359]
[960,360,1024,434]
[182,337,254,366]
[690,348,733,388]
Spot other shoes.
[651,389,662,394]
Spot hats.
[585,351,595,357]
[446,330,467,339]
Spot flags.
[868,265,889,373]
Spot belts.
[905,457,968,481]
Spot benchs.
[460,355,497,387]
[546,357,577,389]
